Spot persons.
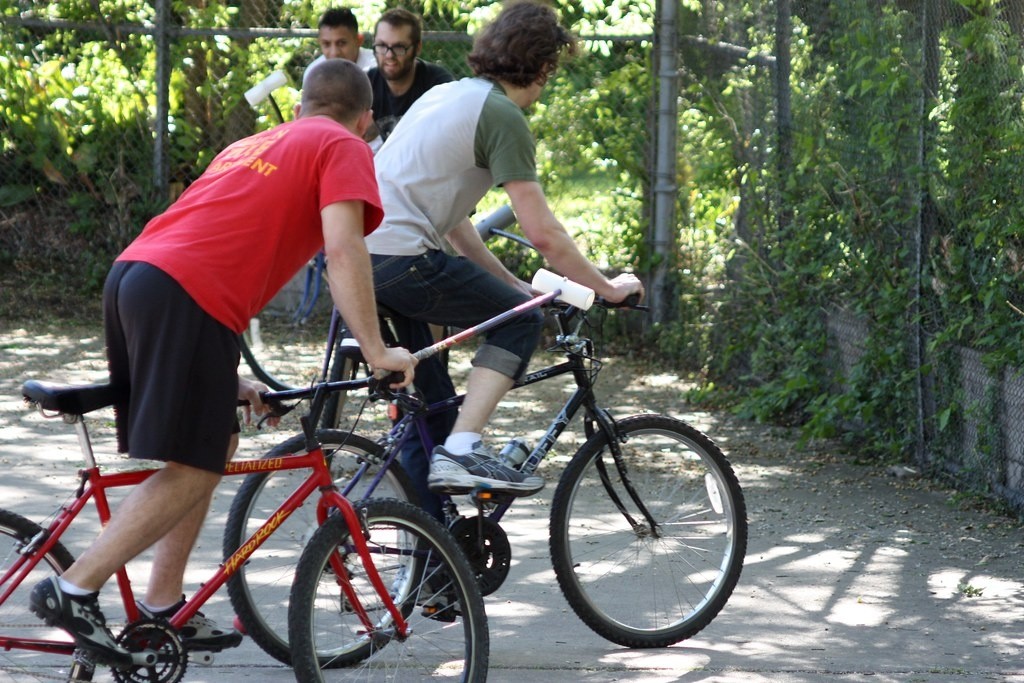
[299,8,383,158]
[364,0,644,613]
[27,58,416,663]
[366,10,453,145]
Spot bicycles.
[0,256,750,683]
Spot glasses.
[373,43,414,55]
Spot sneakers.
[29,576,133,666]
[390,568,462,613]
[427,440,545,497]
[135,594,243,653]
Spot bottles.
[470,436,535,510]
